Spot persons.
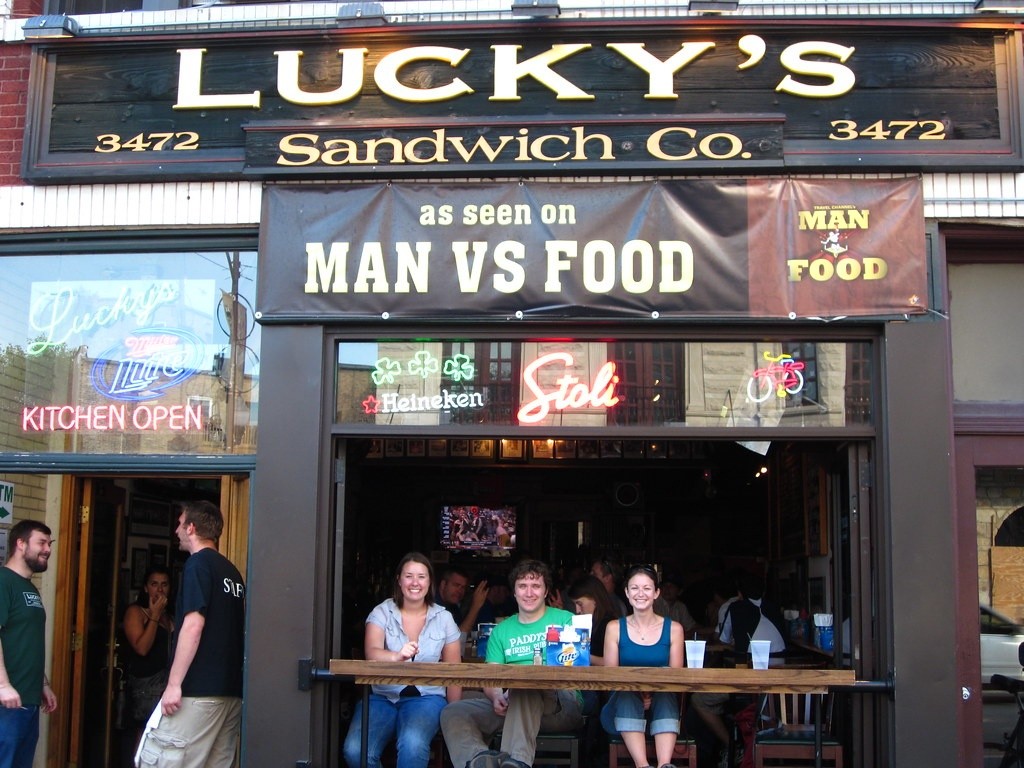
[602,564,685,768]
[118,565,177,768]
[0,519,58,768]
[563,572,626,714]
[341,551,462,768]
[432,557,807,768]
[435,558,579,768]
[133,500,247,768]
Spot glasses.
[631,565,656,578]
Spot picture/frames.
[127,494,185,601]
[362,439,713,458]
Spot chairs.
[609,692,697,768]
[754,665,843,768]
[494,715,592,768]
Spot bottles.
[472,640,477,657]
[534,648,543,665]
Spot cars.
[979,602,1024,692]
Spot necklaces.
[642,638,645,640]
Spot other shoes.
[500,758,530,768]
[718,748,745,767]
[469,750,510,768]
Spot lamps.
[22,14,78,40]
[511,0,562,18]
[974,0,1024,12]
[688,0,739,12]
[336,3,389,28]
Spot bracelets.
[42,682,50,685]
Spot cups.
[750,640,771,669]
[460,632,467,659]
[685,640,706,668]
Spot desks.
[328,659,855,768]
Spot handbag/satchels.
[127,668,167,726]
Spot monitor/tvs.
[435,501,519,562]
